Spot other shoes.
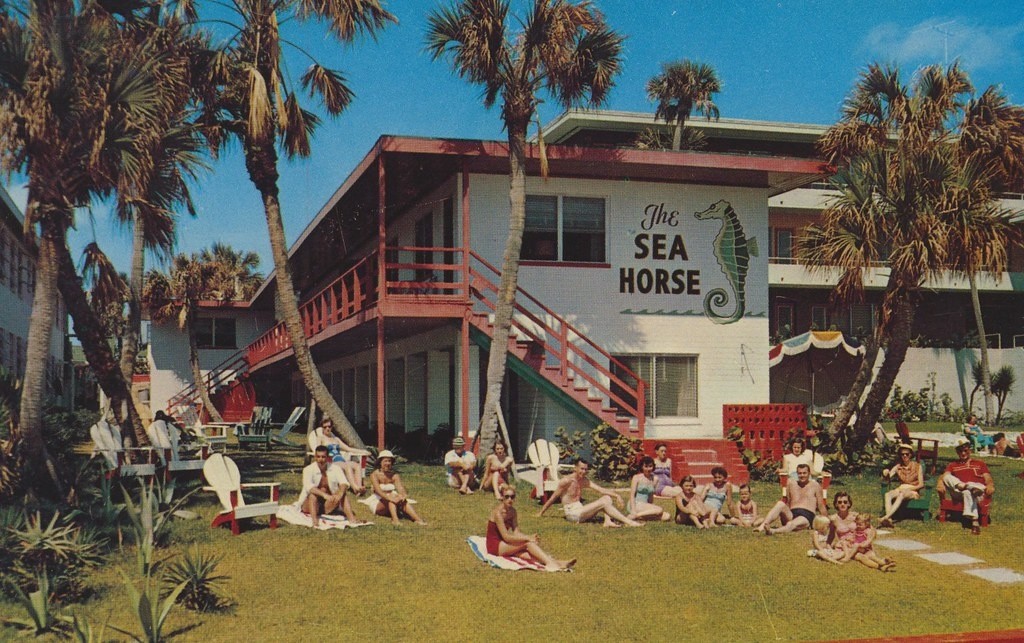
[972,526,980,535]
[467,489,474,495]
[970,488,983,497]
[459,484,466,495]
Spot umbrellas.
[768,327,867,431]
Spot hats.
[453,438,465,447]
[376,450,398,464]
[954,439,970,449]
[897,444,914,459]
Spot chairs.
[89,422,156,489]
[894,436,940,474]
[529,440,565,504]
[307,428,371,482]
[960,424,994,453]
[147,420,208,484]
[263,408,309,450]
[169,405,229,457]
[781,452,832,510]
[199,453,279,535]
[878,459,936,525]
[235,407,273,446]
[939,491,992,526]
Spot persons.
[300,414,1024,570]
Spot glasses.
[504,495,515,500]
[837,500,848,504]
[901,452,910,456]
[323,426,332,429]
